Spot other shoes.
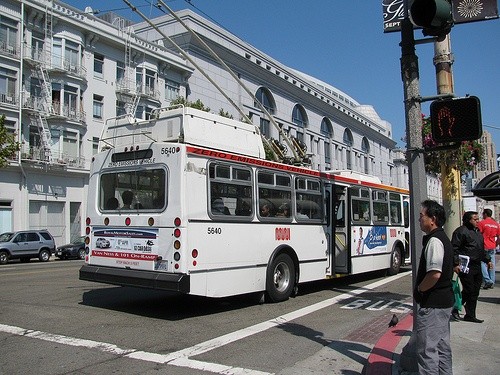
[464,315,484,323]
[483,282,493,290]
[451,310,463,320]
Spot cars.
[54,236,86,261]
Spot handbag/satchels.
[451,275,462,311]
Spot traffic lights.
[429,93,483,145]
[406,0,455,38]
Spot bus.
[77,102,413,305]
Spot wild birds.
[387,314,398,328]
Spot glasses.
[472,218,479,222]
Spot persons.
[212,191,251,216]
[107,191,162,210]
[452,211,494,323]
[413,199,455,375]
[478,209,500,289]
[260,203,309,219]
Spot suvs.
[0,228,57,265]
